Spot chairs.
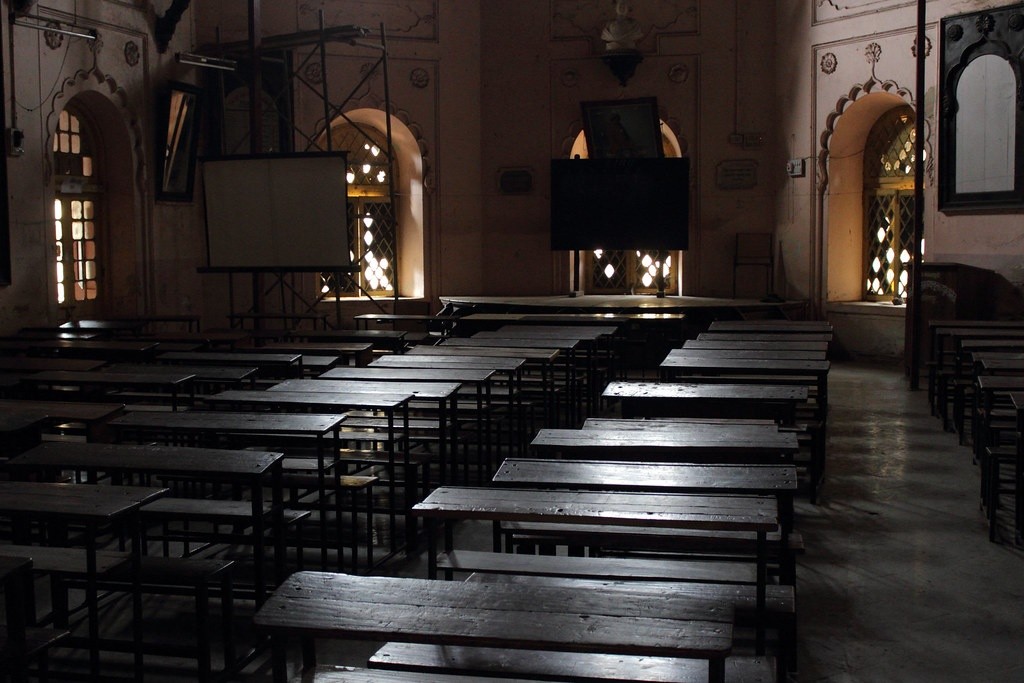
[733,231,775,296]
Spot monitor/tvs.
[551,157,689,251]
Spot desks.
[317,365,496,483]
[491,457,797,533]
[0,398,126,424]
[366,353,526,457]
[903,261,993,378]
[249,567,735,683]
[411,485,779,656]
[473,329,604,344]
[600,379,809,423]
[402,344,560,426]
[975,375,1024,498]
[107,412,347,570]
[6,441,284,610]
[529,428,798,464]
[439,337,579,355]
[267,377,464,483]
[201,389,415,539]
[1009,392,1024,544]
[0,477,171,683]
[981,360,1024,376]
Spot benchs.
[0,332,1023,682]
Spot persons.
[601,0,643,51]
[606,112,642,158]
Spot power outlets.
[786,159,804,176]
[8,128,24,155]
